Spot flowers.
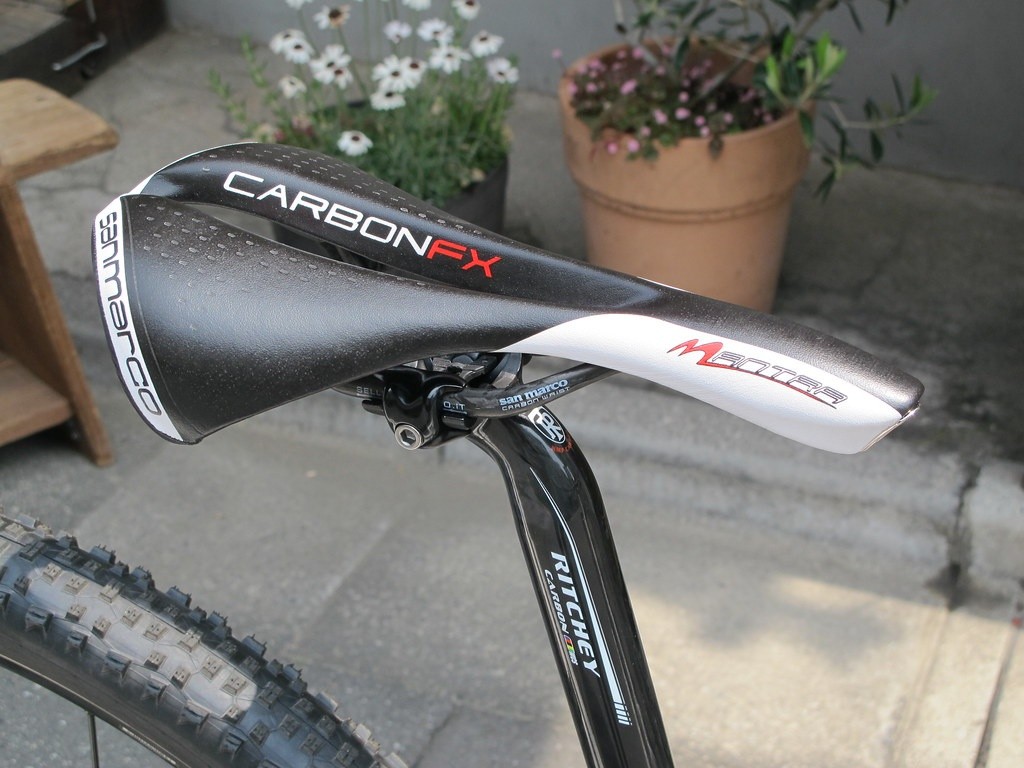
[552,0,931,205]
[206,0,520,211]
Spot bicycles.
[0,142,926,768]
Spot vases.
[556,37,816,316]
[264,101,509,235]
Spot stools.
[0,78,120,469]
